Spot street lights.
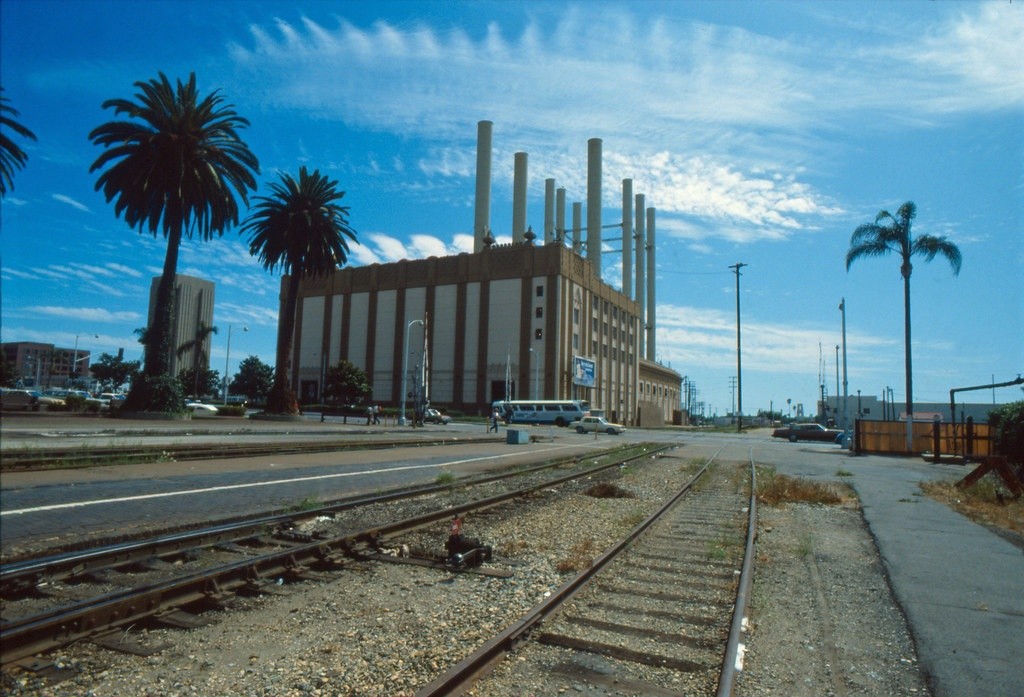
[728,262,747,431]
[222,323,249,406]
[398,319,424,426]
[312,349,327,404]
[529,346,539,401]
[72,333,100,374]
[835,345,840,431]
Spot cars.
[404,408,452,425]
[771,423,846,443]
[567,415,628,435]
[835,428,854,444]
[0,381,220,418]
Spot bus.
[491,400,592,427]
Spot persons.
[490,408,504,433]
[365,403,381,426]
